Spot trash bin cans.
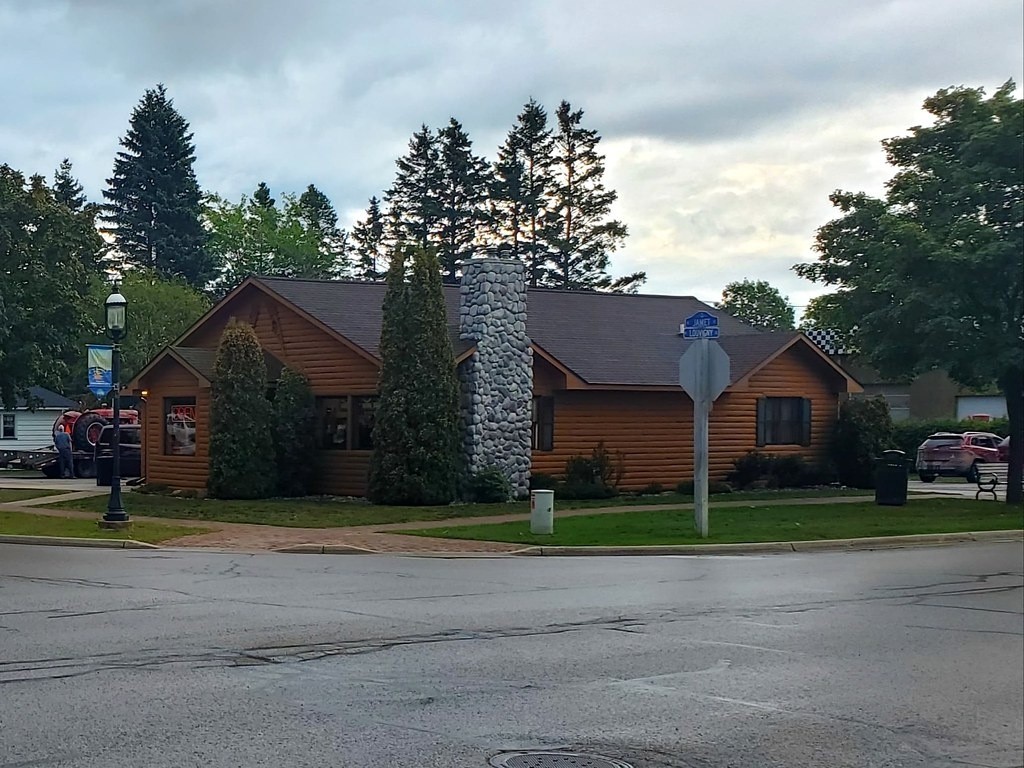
[96,452,114,485]
[532,490,553,534]
[874,450,908,504]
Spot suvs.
[92,424,141,478]
[997,435,1013,461]
[916,430,1004,483]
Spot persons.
[55,425,78,479]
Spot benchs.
[973,460,1009,503]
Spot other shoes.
[61,476,65,478]
[70,476,77,478]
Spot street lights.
[99,280,131,522]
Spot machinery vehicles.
[0,408,142,478]
